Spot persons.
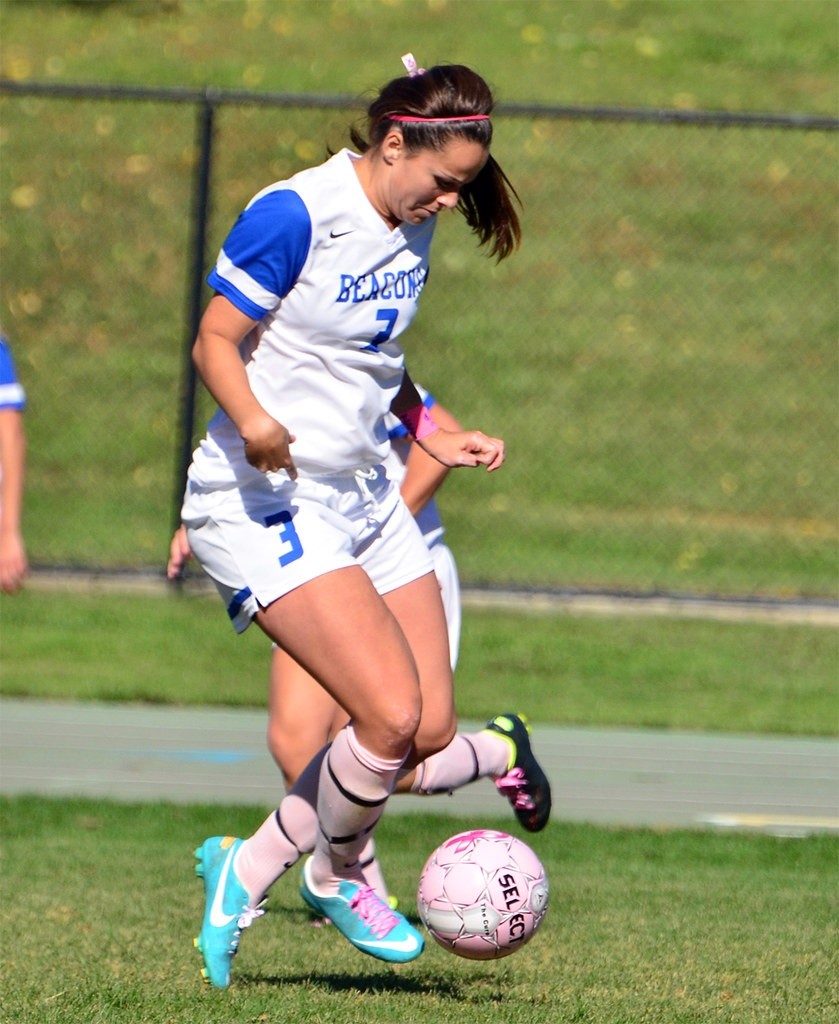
[0,338,31,595]
[183,52,524,991]
[166,380,552,928]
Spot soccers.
[415,827,551,963]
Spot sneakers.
[310,895,398,927]
[299,855,425,963]
[481,712,552,833]
[193,836,270,989]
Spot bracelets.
[397,403,439,441]
